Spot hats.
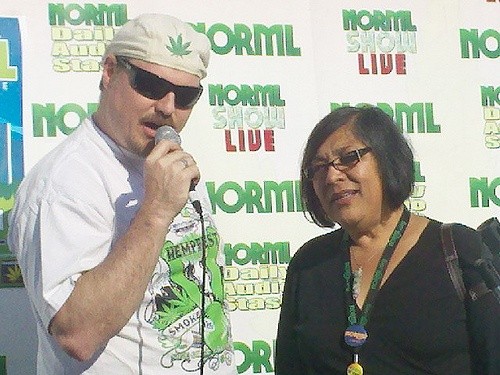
[102,13,212,79]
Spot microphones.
[153,125,202,216]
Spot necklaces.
[348,235,391,300]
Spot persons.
[6,14,239,375]
[276,105,499,374]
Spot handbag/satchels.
[440,218,500,375]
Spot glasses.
[303,147,370,181]
[119,58,204,108]
